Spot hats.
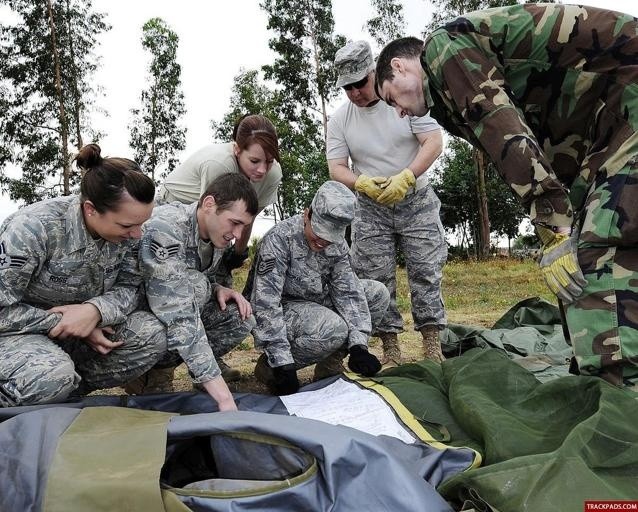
[309,181,360,244]
[334,40,376,88]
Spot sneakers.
[255,353,272,384]
[144,368,175,391]
[190,357,241,382]
[314,353,347,380]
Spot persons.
[326,41,449,371]
[0,143,168,407]
[119,172,257,410]
[373,2,638,390]
[249,179,390,396]
[161,114,284,383]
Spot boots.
[379,332,401,371]
[419,327,447,362]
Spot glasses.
[342,76,370,90]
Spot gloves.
[269,365,302,396]
[354,174,388,200]
[347,348,381,376]
[377,168,416,205]
[530,214,589,304]
